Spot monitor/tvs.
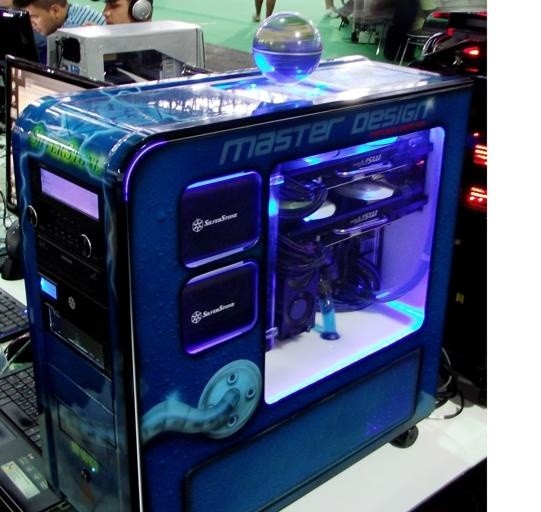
[6,54,117,218]
[0,7,39,87]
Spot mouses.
[7,334,37,366]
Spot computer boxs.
[404,39,486,411]
[12,55,474,512]
[47,17,207,85]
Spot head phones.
[124,1,155,24]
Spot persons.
[0,0,51,65]
[13,0,106,38]
[253,0,276,25]
[81,0,153,25]
[325,0,339,19]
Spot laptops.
[0,361,71,512]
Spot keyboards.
[0,286,30,341]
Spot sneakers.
[323,6,340,18]
